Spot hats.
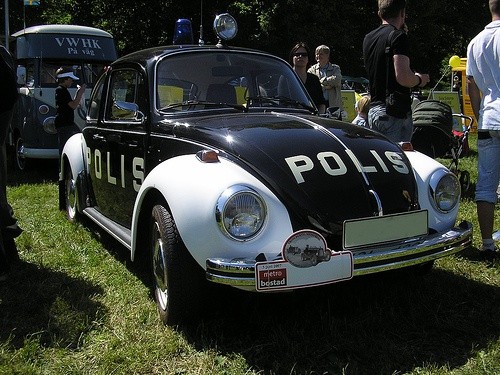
[57,72,80,80]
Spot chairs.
[206,85,237,109]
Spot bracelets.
[416,75,422,85]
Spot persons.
[354,95,371,128]
[466,0,500,258]
[307,45,342,120]
[278,43,326,114]
[54,66,87,157]
[363,0,429,142]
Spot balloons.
[449,56,461,68]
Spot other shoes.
[482,243,494,254]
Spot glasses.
[293,53,307,57]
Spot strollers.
[409,99,472,189]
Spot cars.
[11,23,117,171]
[58,14,475,328]
